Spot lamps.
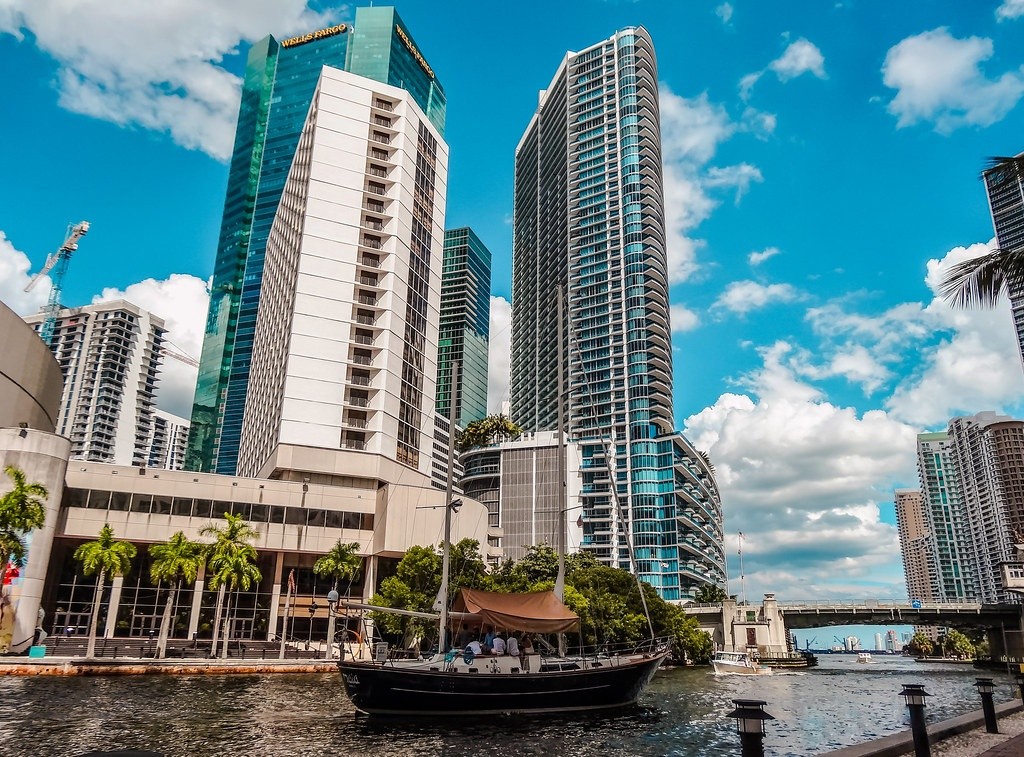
[973,678,999,734]
[452,500,463,513]
[1015,673,1024,706]
[726,699,775,757]
[898,684,934,757]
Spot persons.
[456,622,535,656]
[723,651,758,663]
[903,649,970,660]
[32,601,45,646]
[603,641,615,657]
[0,596,15,655]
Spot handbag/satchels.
[481,644,492,656]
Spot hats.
[496,632,501,635]
[473,625,478,629]
[521,633,527,640]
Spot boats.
[327,284,680,729]
[857,652,871,662]
[711,650,771,676]
